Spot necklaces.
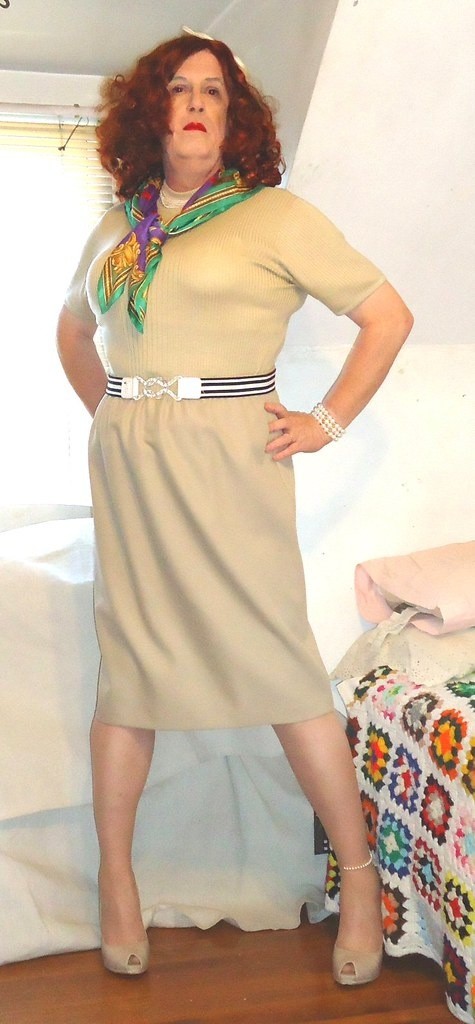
[159,192,186,209]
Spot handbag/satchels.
[337,541,475,678]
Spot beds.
[318,660,475,1022]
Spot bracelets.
[312,403,345,440]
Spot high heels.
[335,901,383,984]
[91,871,151,972]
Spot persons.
[56,37,413,986]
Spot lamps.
[0,70,113,125]
